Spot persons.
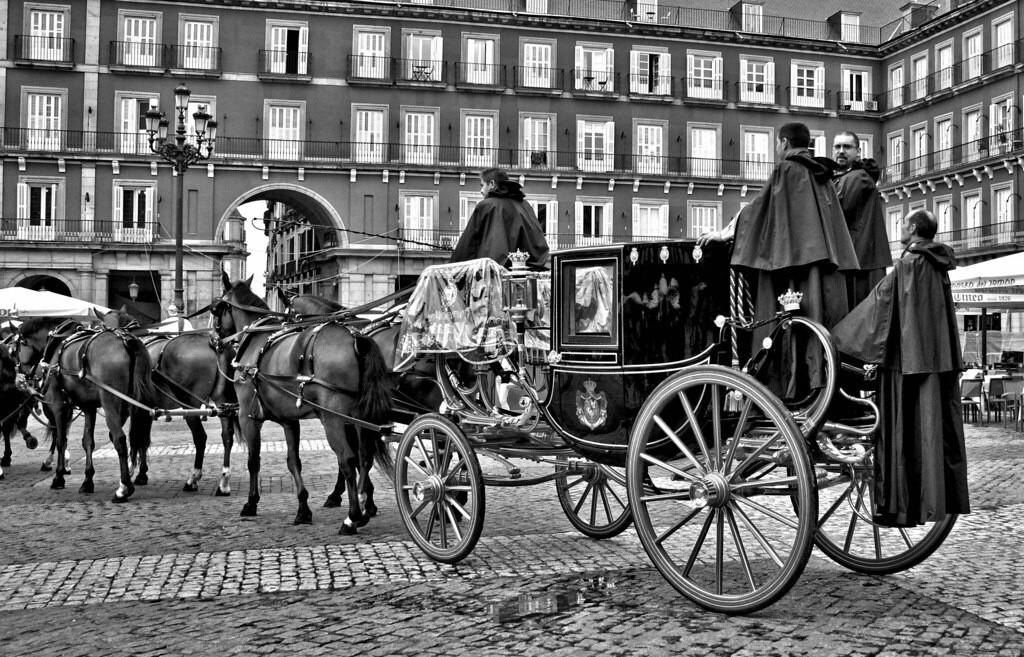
[449,167,549,268]
[829,210,971,528]
[695,123,860,404]
[829,131,893,314]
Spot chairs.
[961,379,985,427]
[662,11,671,24]
[600,77,609,90]
[983,378,1004,425]
[1001,375,1024,432]
[411,63,435,81]
[630,8,641,22]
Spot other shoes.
[873,512,925,528]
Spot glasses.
[832,144,858,150]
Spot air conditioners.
[864,100,878,112]
[440,234,461,250]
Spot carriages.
[0,238,961,614]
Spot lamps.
[502,248,538,370]
[128,275,139,303]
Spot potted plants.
[996,122,1008,154]
[977,139,989,159]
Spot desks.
[583,76,597,90]
[413,66,430,81]
[646,12,656,24]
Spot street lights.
[144,81,219,319]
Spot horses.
[207,269,469,536]
[0,305,241,506]
